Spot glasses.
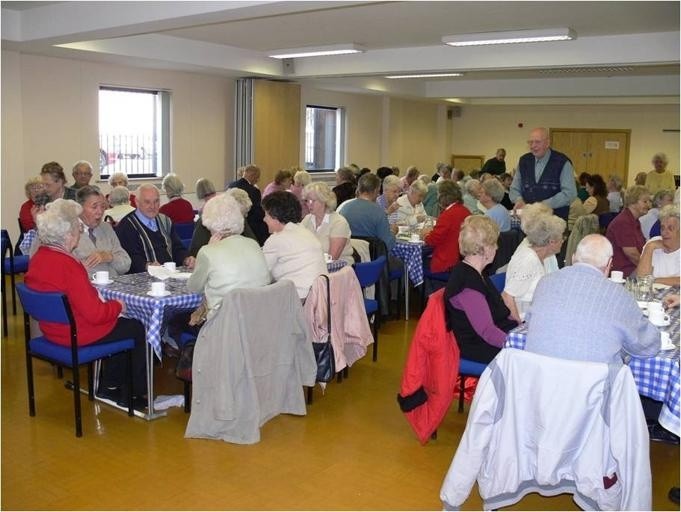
[529,138,547,142]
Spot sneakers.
[97,388,147,407]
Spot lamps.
[264,42,367,59]
[441,28,577,47]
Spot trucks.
[100,129,150,161]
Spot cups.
[93,271,109,282]
[324,253,333,262]
[161,262,177,272]
[398,216,437,243]
[610,271,671,327]
[152,282,166,292]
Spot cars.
[98,130,118,173]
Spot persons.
[16,127,680,505]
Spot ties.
[88,228,96,245]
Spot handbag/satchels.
[312,342,335,382]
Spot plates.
[147,291,171,297]
[92,280,115,285]
